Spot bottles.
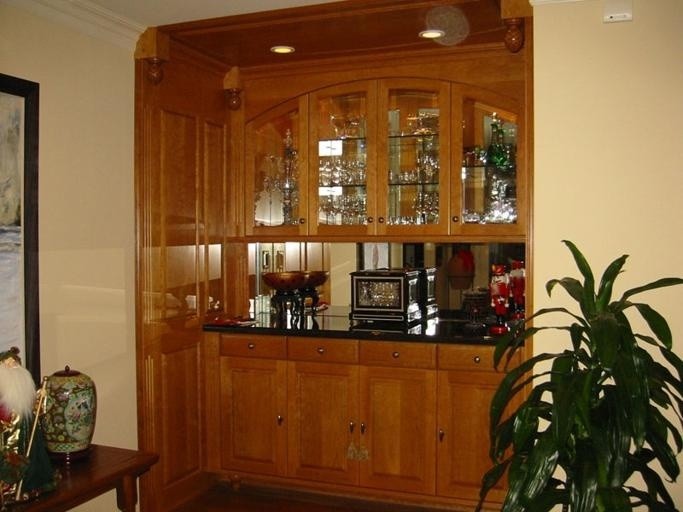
[486,112,504,166]
[496,118,508,168]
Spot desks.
[0,442,159,512]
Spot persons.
[508,256,525,320]
[488,262,511,335]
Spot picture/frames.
[357,243,392,270]
[0,72,40,390]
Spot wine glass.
[411,192,441,224]
[318,154,369,226]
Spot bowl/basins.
[259,270,304,295]
[300,271,331,294]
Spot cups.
[328,113,362,139]
[387,170,417,185]
[389,215,407,226]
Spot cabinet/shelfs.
[202,328,287,489]
[287,332,437,508]
[452,82,529,236]
[243,95,310,237]
[436,336,532,512]
[255,242,329,306]
[308,76,451,235]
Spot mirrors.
[246,243,526,322]
